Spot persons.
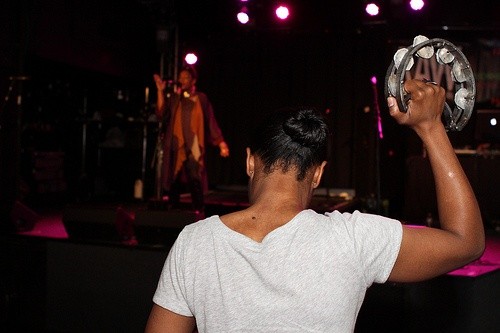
[144,79,486,333]
[153,65,230,208]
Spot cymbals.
[383,35,477,134]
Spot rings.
[425,82,437,84]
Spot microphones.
[169,80,181,86]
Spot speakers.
[62,204,134,242]
[136,207,203,249]
[0,201,39,234]
